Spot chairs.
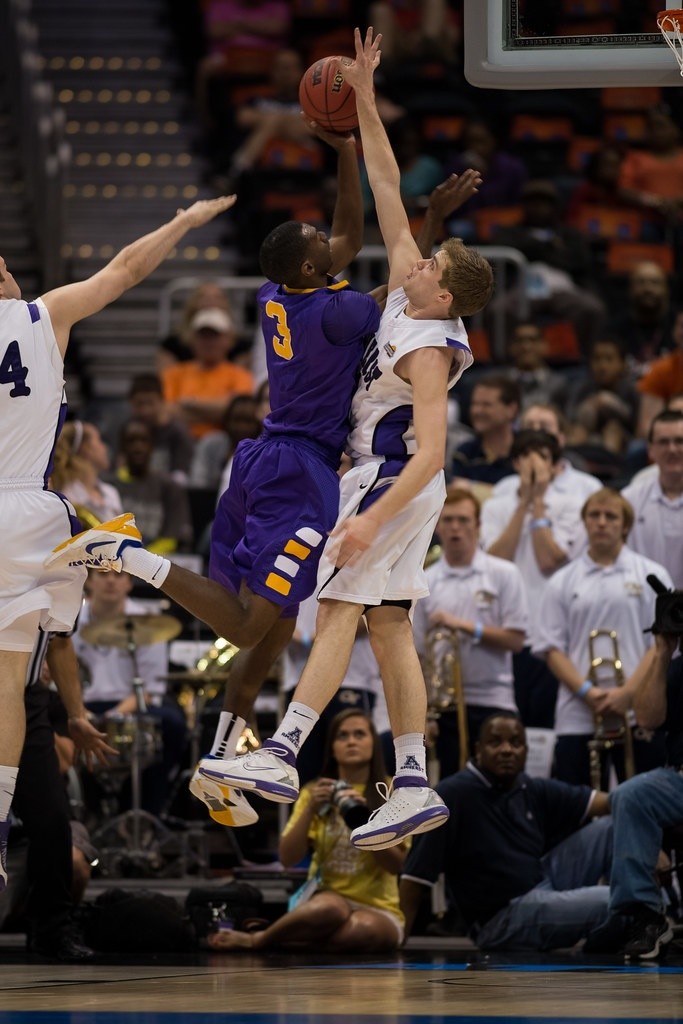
[74,713,310,966]
[194,0,683,396]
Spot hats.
[192,309,234,335]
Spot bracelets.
[577,680,592,699]
[530,518,552,531]
[472,622,484,646]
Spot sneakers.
[0,821,10,891]
[189,754,258,826]
[199,738,299,803]
[350,775,450,851]
[42,512,142,574]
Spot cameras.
[318,780,373,830]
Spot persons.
[1,196,235,963]
[41,1,683,963]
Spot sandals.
[208,930,257,953]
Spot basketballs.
[299,55,359,131]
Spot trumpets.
[578,629,637,786]
[420,622,471,780]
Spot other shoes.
[623,920,674,961]
[25,936,97,959]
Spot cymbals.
[78,609,186,650]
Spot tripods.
[88,626,245,880]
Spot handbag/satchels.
[185,884,264,923]
[85,888,199,964]
